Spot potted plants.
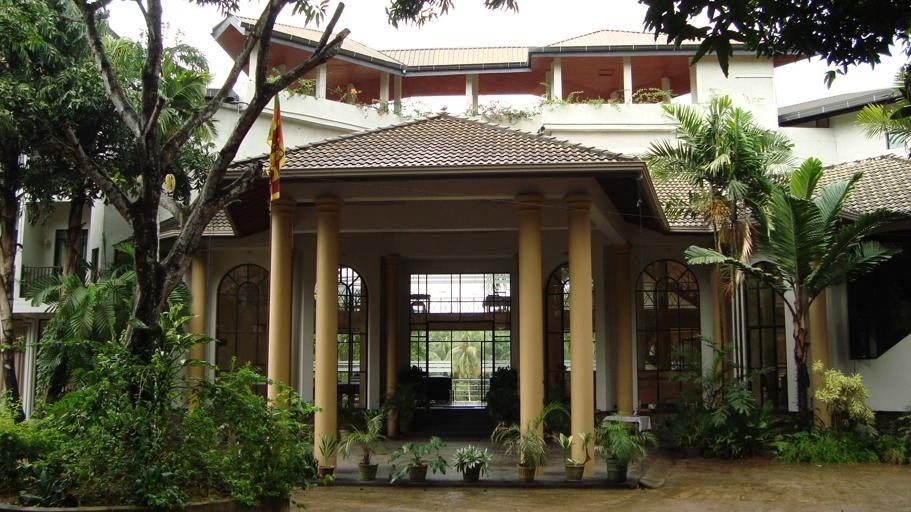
[553,432,594,482]
[317,430,339,483]
[452,444,493,482]
[388,436,452,483]
[658,386,722,459]
[492,401,571,481]
[339,407,390,482]
[595,412,658,482]
